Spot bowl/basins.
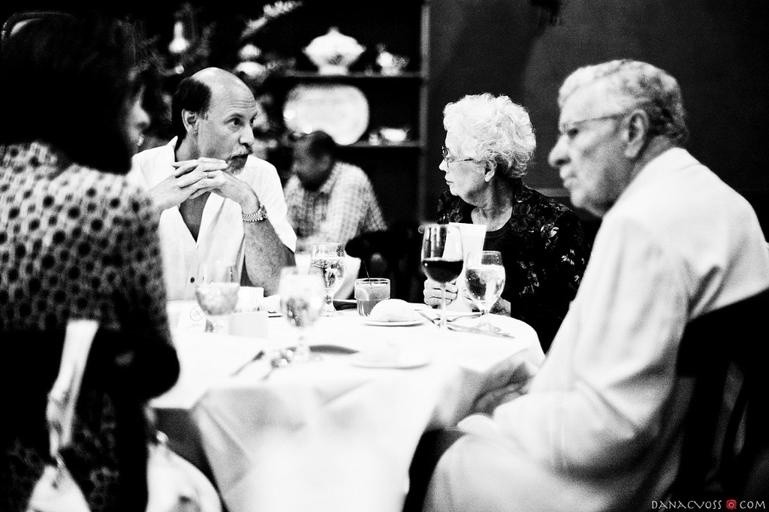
[301,26,364,75]
[294,254,361,300]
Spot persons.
[126,66,295,303]
[415,94,605,358]
[281,129,385,268]
[0,11,181,512]
[408,61,767,512]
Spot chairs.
[345,228,411,298]
[629,285,768,512]
[1,291,153,512]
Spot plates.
[367,321,425,327]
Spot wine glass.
[421,223,464,332]
[309,243,345,314]
[465,251,506,332]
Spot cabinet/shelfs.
[207,0,432,233]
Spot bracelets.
[240,204,267,221]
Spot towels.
[187,362,447,512]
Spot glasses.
[441,146,476,163]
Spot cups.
[194,265,324,327]
[355,278,392,315]
[368,128,410,147]
